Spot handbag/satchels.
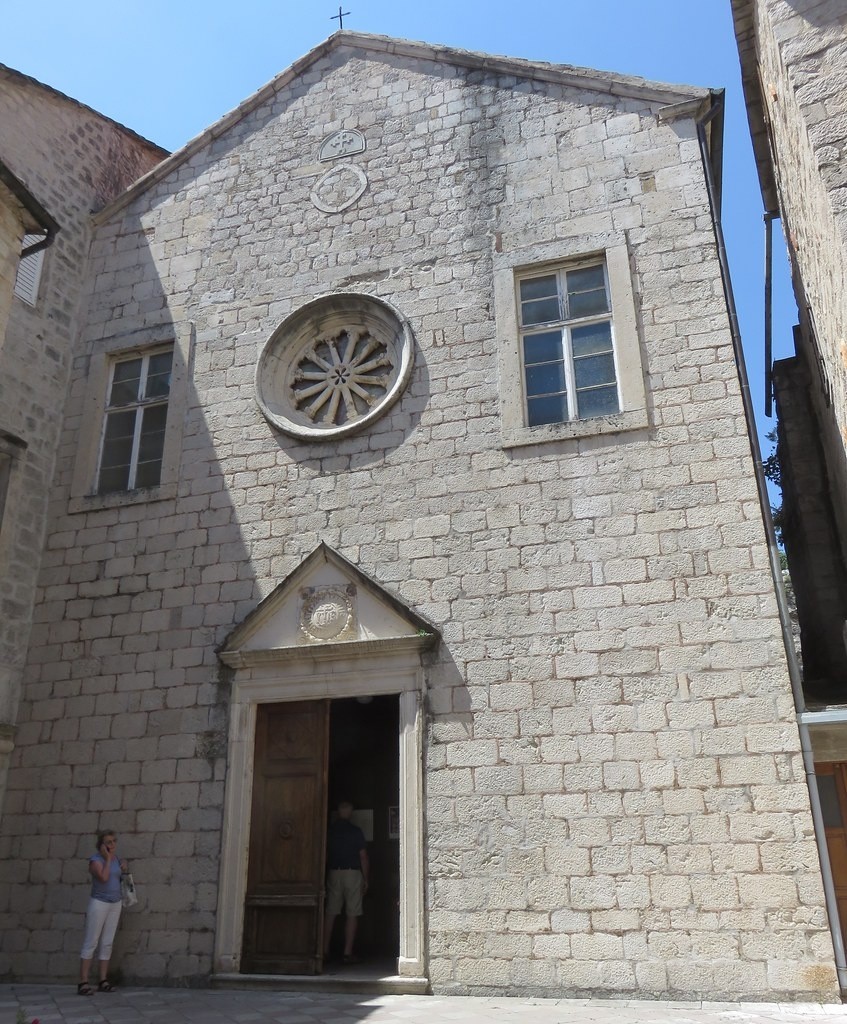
[120,873,138,908]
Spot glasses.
[104,839,116,844]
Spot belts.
[325,864,361,870]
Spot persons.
[78,830,127,996]
[323,801,368,966]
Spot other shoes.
[343,954,366,963]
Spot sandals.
[97,980,118,992]
[78,982,93,995]
